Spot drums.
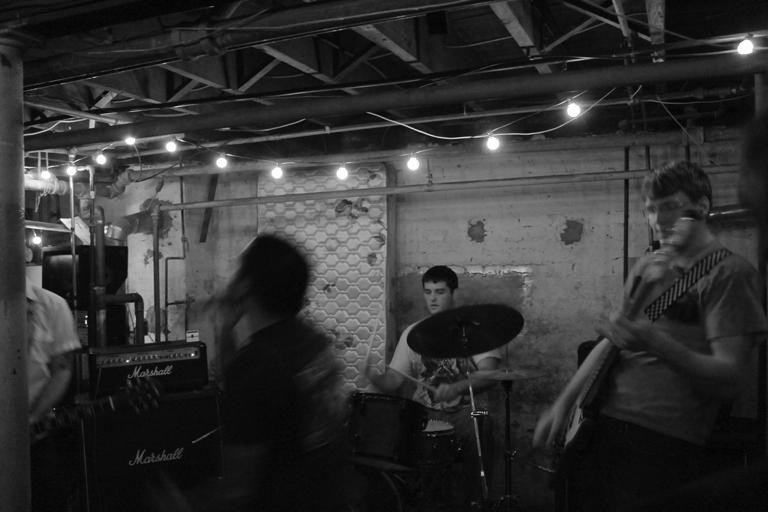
[413,419,457,464]
[341,466,417,512]
[346,391,428,475]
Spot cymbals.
[406,303,525,359]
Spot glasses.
[641,199,692,218]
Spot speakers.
[31,391,224,512]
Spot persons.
[357,265,504,512]
[530,158,766,512]
[24,267,81,512]
[200,228,359,512]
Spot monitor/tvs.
[41,245,128,311]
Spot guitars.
[536,208,699,487]
